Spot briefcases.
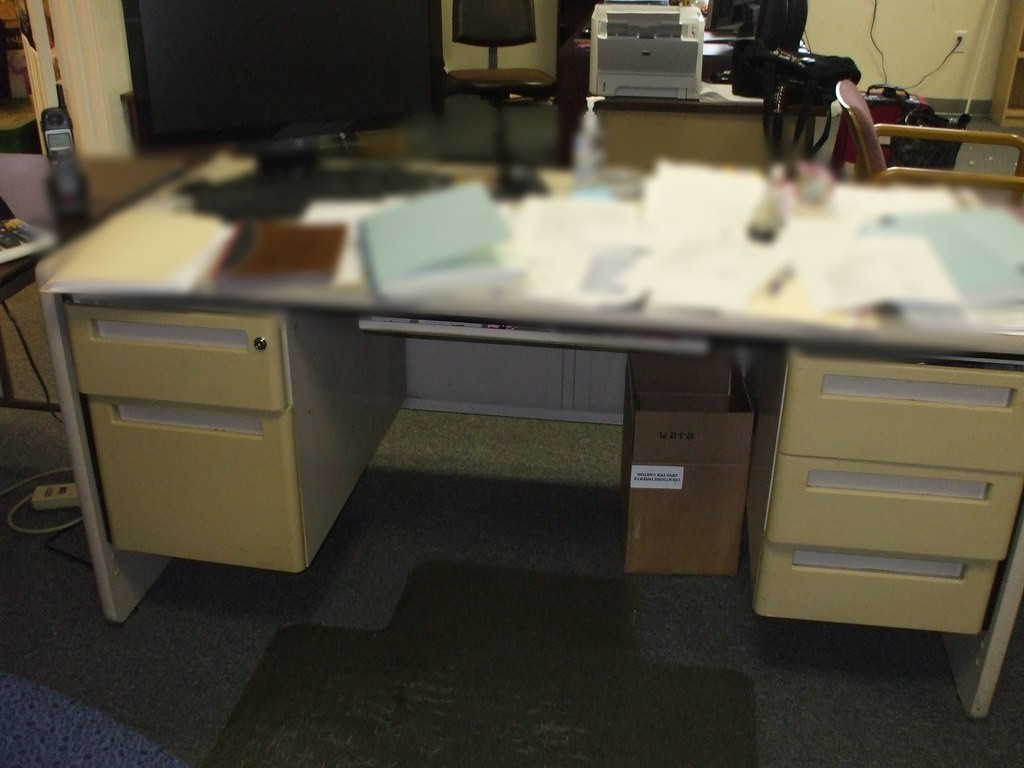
[829,84,934,171]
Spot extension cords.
[31,483,81,510]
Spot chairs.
[835,79,1024,210]
[447,0,557,107]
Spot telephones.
[39,108,84,230]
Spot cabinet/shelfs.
[988,0,1024,128]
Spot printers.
[590,4,705,100]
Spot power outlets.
[951,30,968,53]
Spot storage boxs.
[615,337,755,573]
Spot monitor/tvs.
[121,0,448,218]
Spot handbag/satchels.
[741,49,861,163]
[890,109,972,171]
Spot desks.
[587,83,828,176]
[33,166,1024,722]
